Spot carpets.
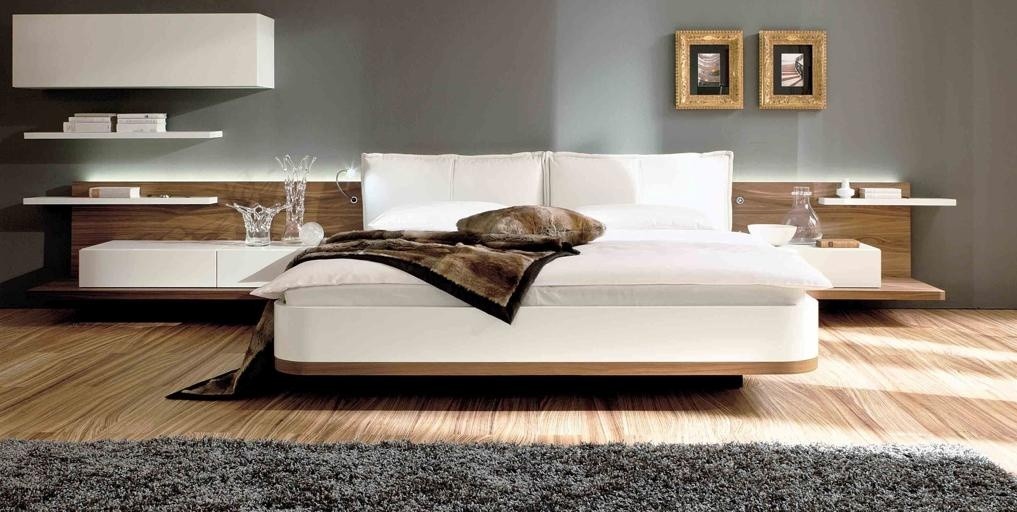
[0,440,1015,512]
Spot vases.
[219,153,318,247]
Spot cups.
[275,155,317,243]
[299,222,325,247]
[230,201,292,247]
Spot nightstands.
[789,236,882,290]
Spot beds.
[274,150,818,399]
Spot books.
[88,186,140,199]
[828,240,859,248]
[816,238,857,248]
[62,111,167,133]
[859,187,902,198]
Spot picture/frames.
[672,28,829,113]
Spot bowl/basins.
[747,222,797,246]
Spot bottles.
[835,178,854,199]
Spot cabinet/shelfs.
[77,236,300,291]
